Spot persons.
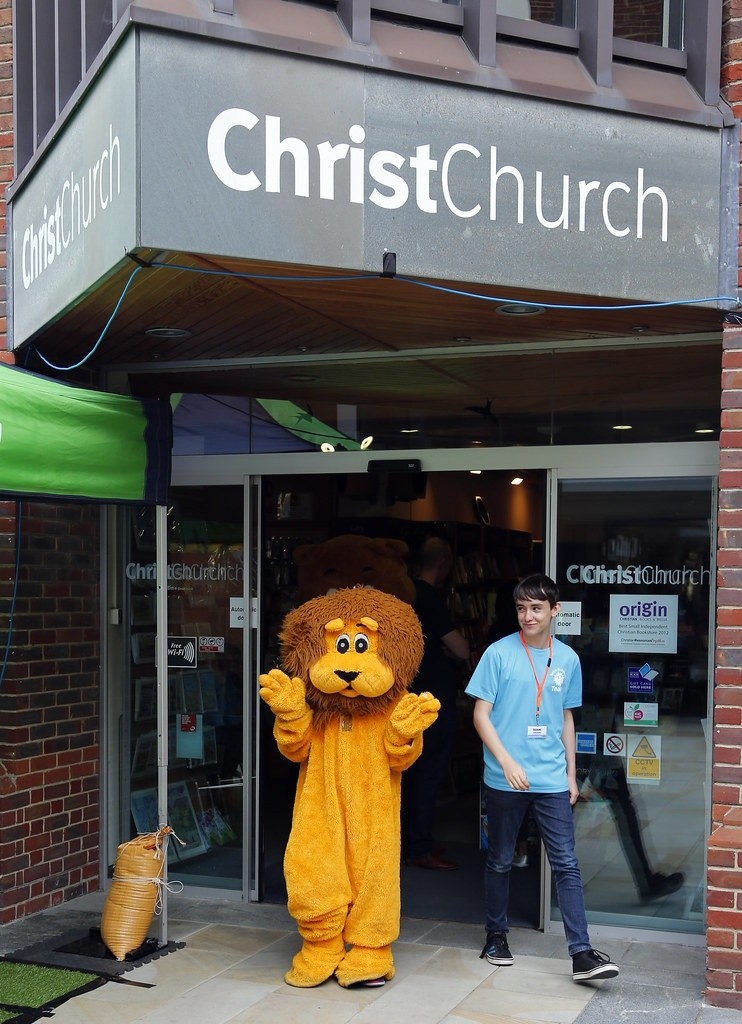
[465,574,620,981]
[411,537,470,871]
[552,603,684,904]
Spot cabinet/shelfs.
[431,520,533,760]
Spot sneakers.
[479,931,514,965]
[572,949,618,980]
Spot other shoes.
[510,855,528,868]
[639,872,684,902]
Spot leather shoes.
[406,853,459,871]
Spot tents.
[0,359,172,947]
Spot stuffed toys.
[292,536,416,605]
[258,587,442,986]
